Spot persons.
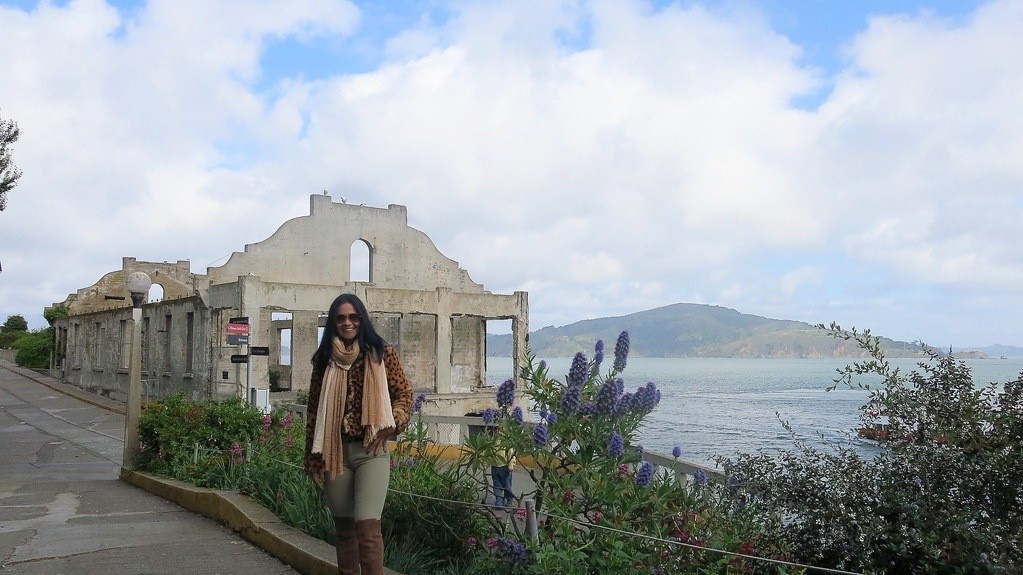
[303,294,415,575]
[482,424,516,510]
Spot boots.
[355,519,384,575]
[331,516,360,575]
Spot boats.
[857,407,950,447]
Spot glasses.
[334,313,362,323]
